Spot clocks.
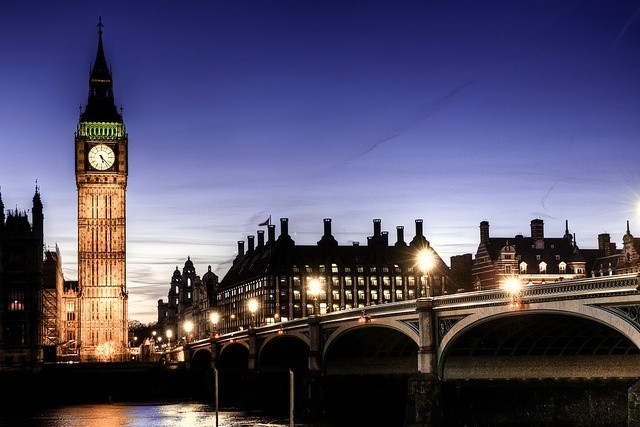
[87,144,116,171]
